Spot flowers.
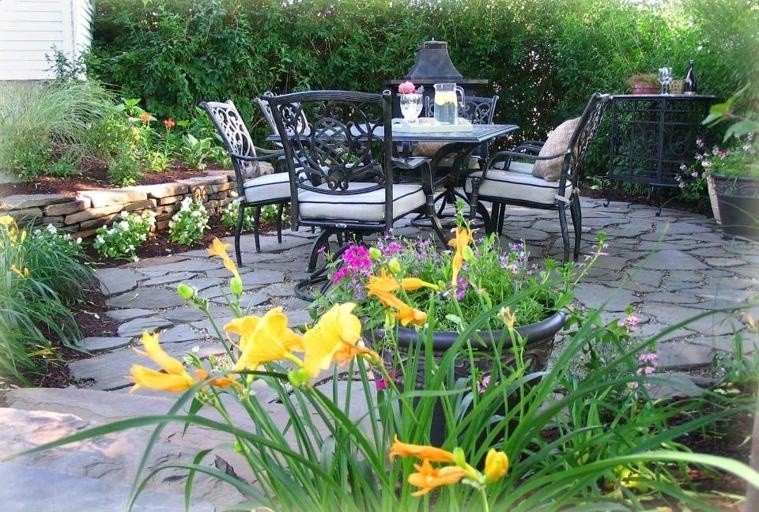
[306,235,611,393]
[666,130,759,196]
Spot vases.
[713,175,759,236]
[352,303,567,445]
[706,174,722,225]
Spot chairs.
[256,94,353,242]
[263,89,452,274]
[199,94,331,264]
[410,90,498,229]
[467,91,614,271]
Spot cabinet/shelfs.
[603,92,716,218]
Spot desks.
[266,124,529,238]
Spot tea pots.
[433,82,465,125]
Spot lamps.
[406,37,463,113]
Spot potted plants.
[626,71,660,95]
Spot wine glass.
[657,69,667,95]
[662,66,673,95]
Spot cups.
[400,94,423,123]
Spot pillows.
[415,141,446,156]
[532,117,581,185]
